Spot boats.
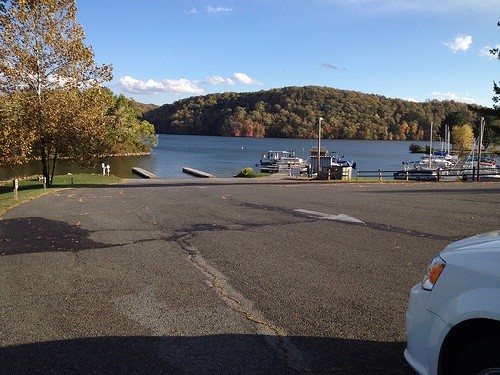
[260,156,308,172]
[260,150,296,165]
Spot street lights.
[318,116,324,178]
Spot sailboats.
[393,116,500,181]
